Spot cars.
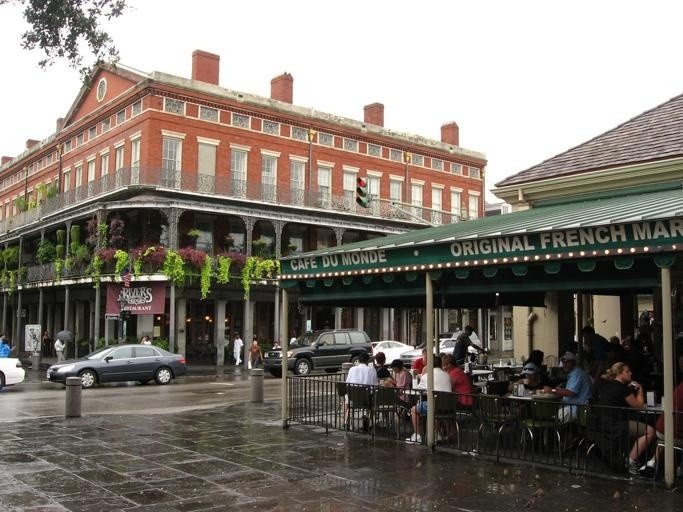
[45,341,190,390]
[370,329,483,365]
[0,356,27,393]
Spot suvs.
[262,325,372,380]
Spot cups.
[511,358,516,366]
[499,359,503,366]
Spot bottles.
[517,384,525,397]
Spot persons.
[0,338,16,358]
[342,312,682,481]
[140,336,145,345]
[54,338,66,363]
[232,334,244,367]
[42,330,53,359]
[144,336,151,344]
[0,333,6,347]
[289,335,297,345]
[251,340,259,368]
[272,342,282,350]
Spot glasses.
[524,368,537,372]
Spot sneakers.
[404,436,422,445]
[638,459,655,474]
[623,457,640,475]
[342,424,350,430]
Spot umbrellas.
[56,330,74,340]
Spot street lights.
[117,291,128,339]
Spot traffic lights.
[355,175,370,210]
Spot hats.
[560,351,577,362]
[520,362,538,375]
